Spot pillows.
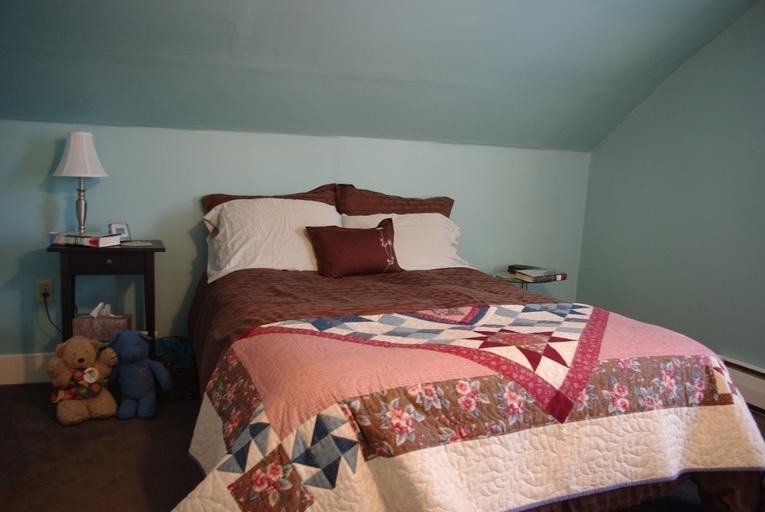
[200,180,337,208]
[306,218,406,276]
[342,213,478,276]
[337,181,455,217]
[201,198,350,286]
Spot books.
[47,230,122,249]
[513,264,568,284]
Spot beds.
[170,265,765,512]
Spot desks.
[47,237,168,385]
[496,265,556,291]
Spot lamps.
[51,131,111,232]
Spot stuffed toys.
[45,334,121,426]
[106,330,174,422]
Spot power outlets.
[35,278,55,303]
